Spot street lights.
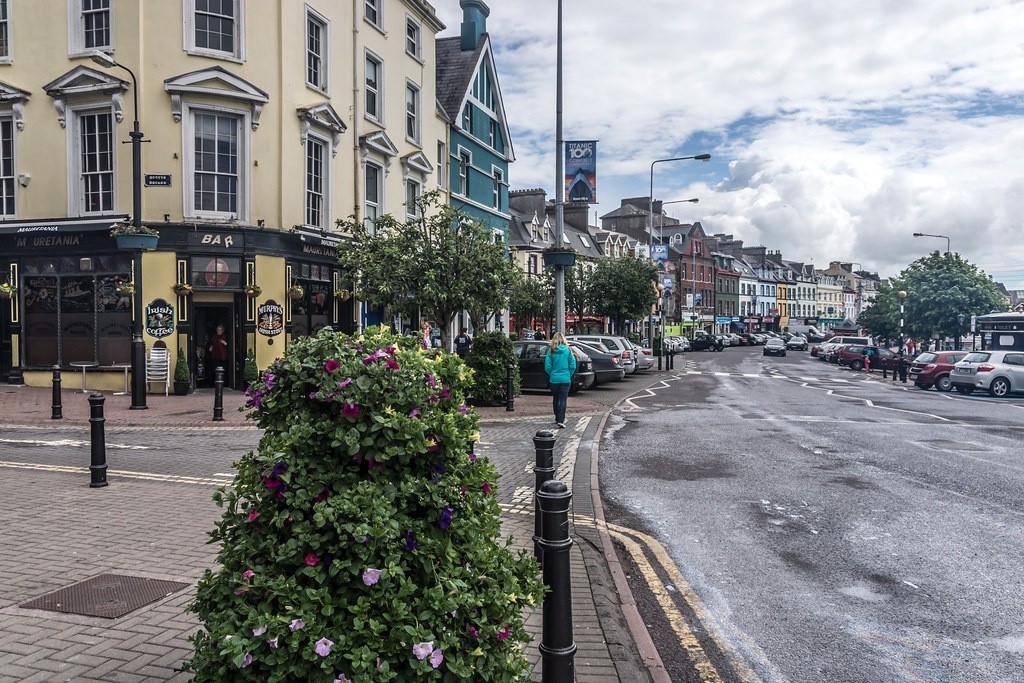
[958,314,965,349]
[659,198,699,354]
[899,290,906,355]
[648,154,712,346]
[91,49,146,411]
[913,233,949,350]
[692,237,721,325]
[837,261,861,336]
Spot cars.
[642,336,690,353]
[717,330,808,347]
[514,339,594,396]
[540,335,655,375]
[811,335,874,365]
[838,345,911,375]
[948,350,1024,397]
[763,339,787,356]
[787,337,808,351]
[517,340,625,387]
[909,351,982,392]
[688,334,724,352]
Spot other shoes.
[557,422,566,428]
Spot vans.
[785,325,825,342]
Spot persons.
[535,330,543,340]
[454,327,472,359]
[864,355,870,373]
[209,324,228,386]
[545,332,576,428]
[906,339,931,355]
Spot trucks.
[660,325,709,341]
